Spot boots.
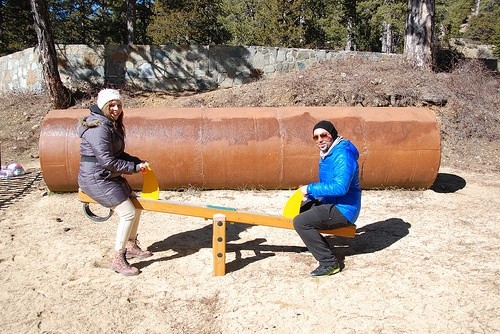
[111,249,140,275]
[126,234,151,257]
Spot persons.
[293,120,362,276]
[76,89,153,276]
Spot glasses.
[313,133,330,142]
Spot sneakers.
[310,264,340,276]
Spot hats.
[313,120,337,142]
[97,88,122,110]
